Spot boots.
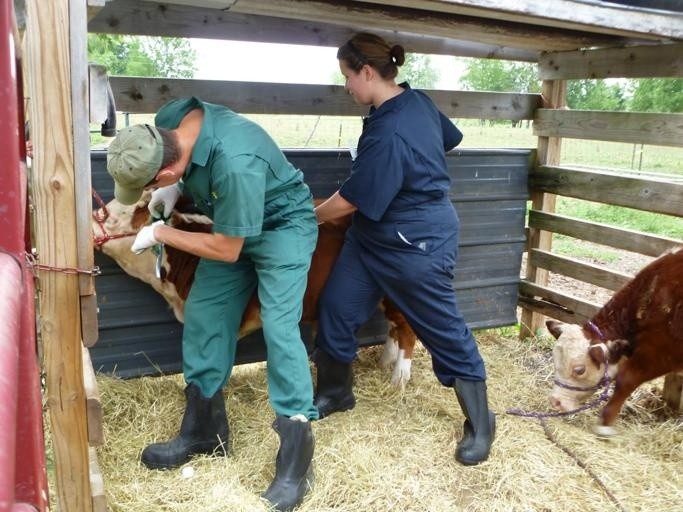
[141,383,228,471]
[454,378,495,466]
[260,416,315,512]
[313,347,356,419]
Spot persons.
[312,31,496,465]
[105,94,320,512]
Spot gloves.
[148,181,183,219]
[131,219,165,255]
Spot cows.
[545,245,683,438]
[92,187,416,397]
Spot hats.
[107,124,164,205]
